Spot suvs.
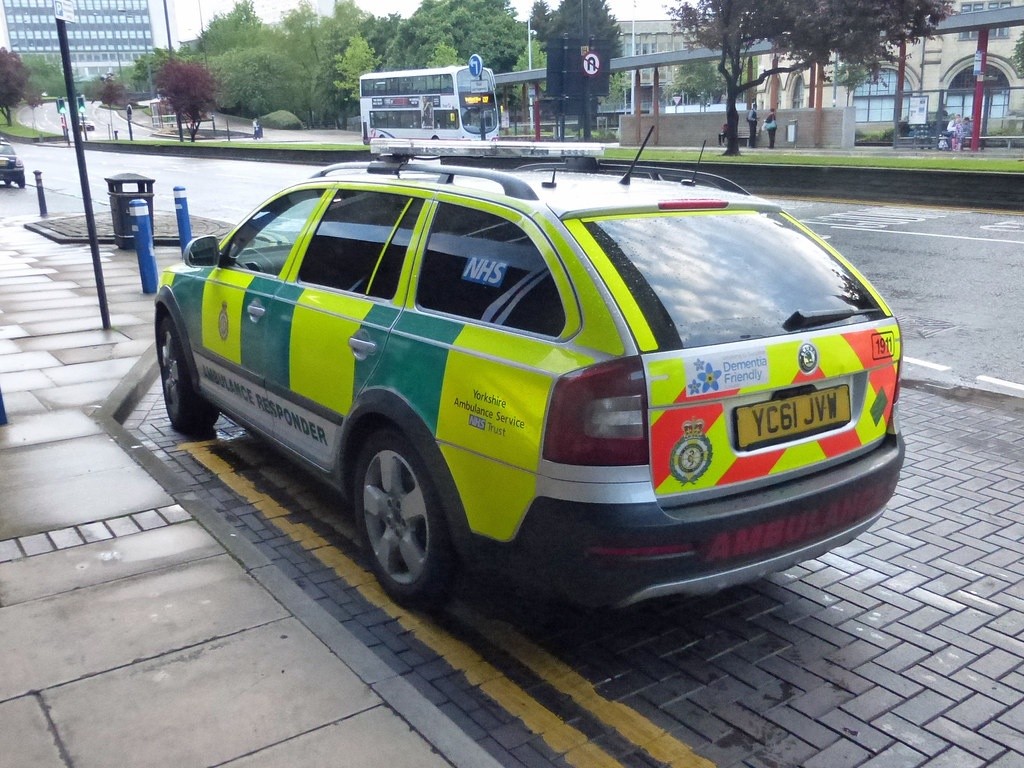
[154,139,906,615]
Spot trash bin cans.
[103,172,156,249]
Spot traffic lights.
[76,97,85,113]
[56,99,66,113]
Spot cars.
[0,137,26,188]
[77,115,94,131]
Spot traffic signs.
[468,54,482,78]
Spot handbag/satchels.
[942,130,953,136]
[765,114,777,129]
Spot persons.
[253,118,260,140]
[945,113,972,152]
[748,104,759,148]
[718,123,729,147]
[764,108,776,149]
[422,101,433,126]
[899,116,915,137]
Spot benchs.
[725,126,760,148]
[940,135,1024,150]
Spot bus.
[360,66,499,145]
